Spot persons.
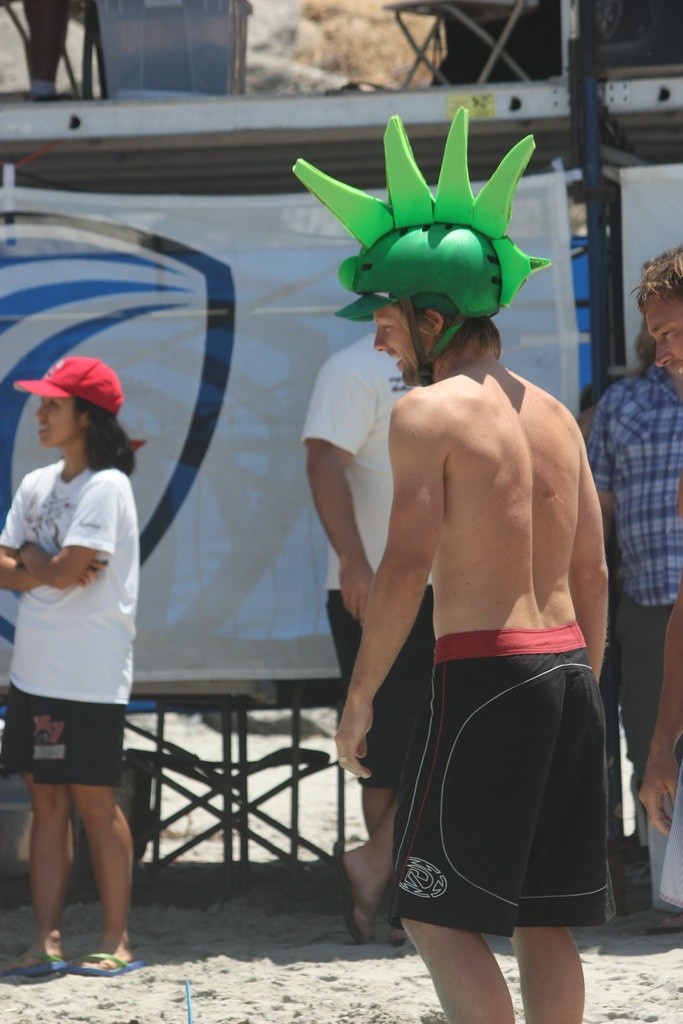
[587,318,683,842]
[334,227,617,1022]
[301,334,437,946]
[0,357,143,977]
[24,0,72,103]
[621,246,683,935]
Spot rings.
[340,756,347,762]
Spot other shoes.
[644,910,683,933]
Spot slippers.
[334,841,378,945]
[60,952,148,976]
[0,946,72,976]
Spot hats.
[13,356,125,415]
[292,103,551,320]
